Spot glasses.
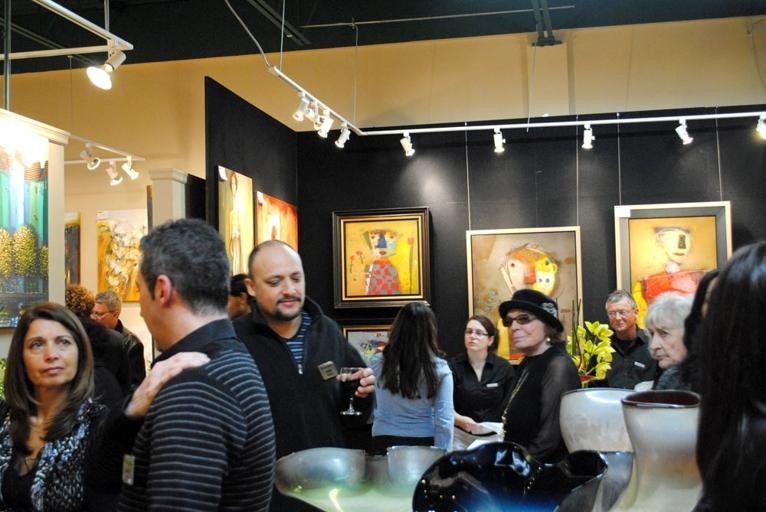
[502,313,539,327]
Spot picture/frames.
[329,205,431,309]
[342,324,393,366]
[614,200,735,332]
[465,225,585,365]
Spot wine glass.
[338,367,362,417]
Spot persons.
[643,292,693,391]
[447,314,513,436]
[103,218,276,512]
[67,274,256,401]
[587,290,662,389]
[498,289,583,460]
[0,304,109,512]
[366,302,455,456]
[691,241,766,511]
[232,240,377,461]
[680,269,722,393]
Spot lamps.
[86,36,126,89]
[399,110,766,160]
[79,143,100,169]
[104,158,142,184]
[292,90,351,150]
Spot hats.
[499,288,564,333]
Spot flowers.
[564,321,617,388]
